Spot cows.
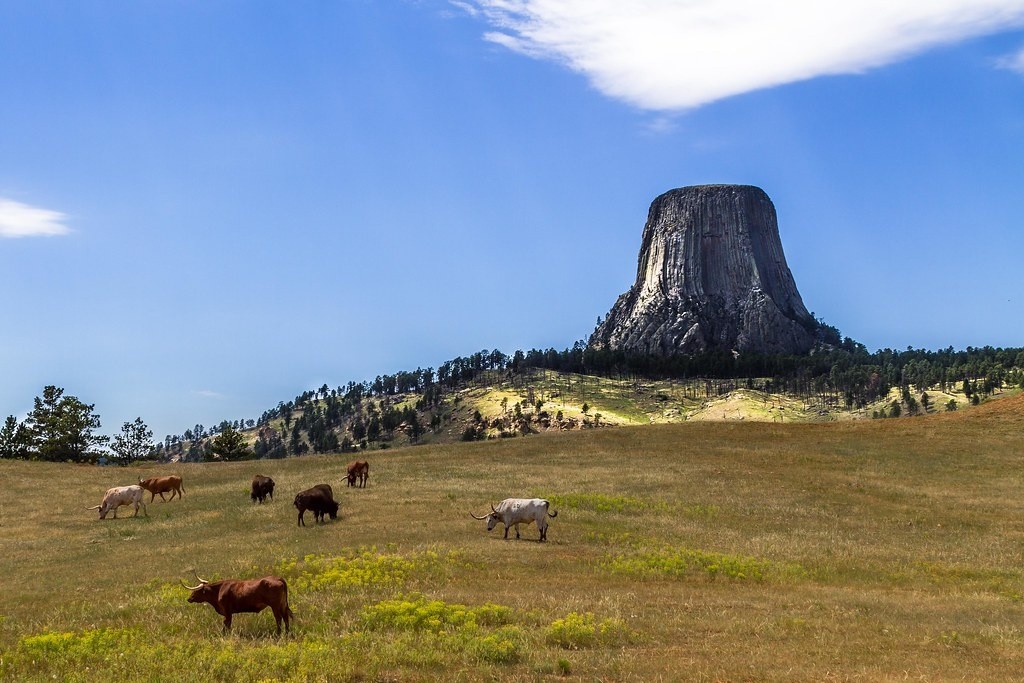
[84,484,148,520]
[138,475,186,504]
[179,568,294,644]
[469,497,559,541]
[339,460,370,488]
[250,474,275,505]
[292,484,341,527]
